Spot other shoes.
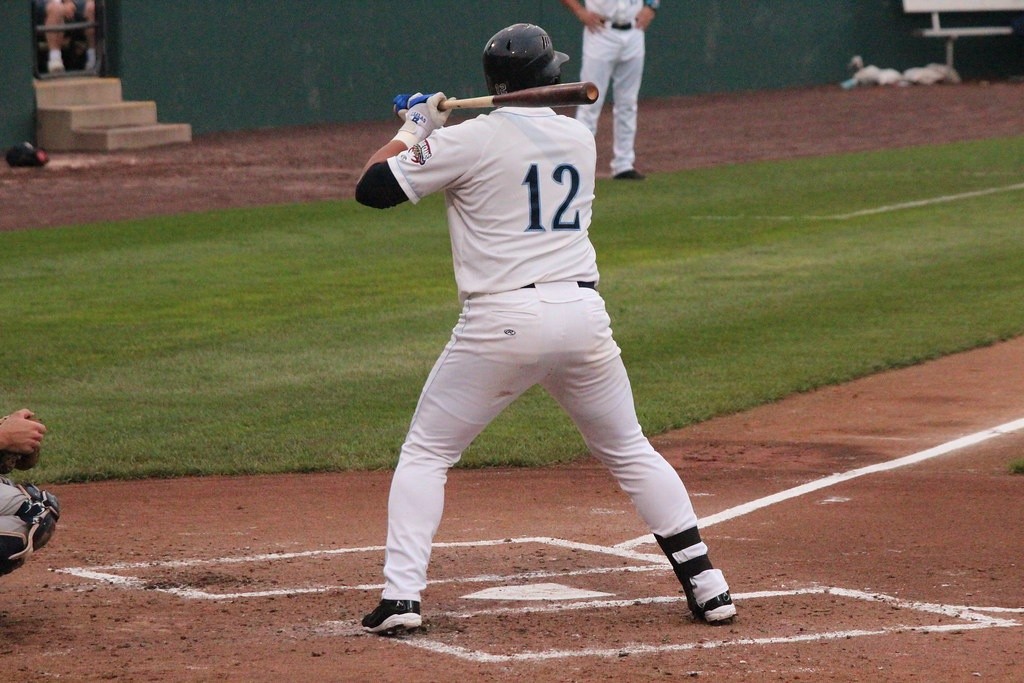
[48,58,64,73]
[86,62,96,72]
[613,169,648,181]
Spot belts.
[522,281,594,289]
[600,18,633,30]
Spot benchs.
[902,0,1024,66]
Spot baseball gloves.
[0,415,43,476]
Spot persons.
[352,21,740,636]
[562,0,662,180]
[36,0,98,76]
[0,406,64,578]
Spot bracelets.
[648,4,657,12]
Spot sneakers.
[695,589,737,622]
[361,599,422,632]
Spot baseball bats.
[393,81,600,110]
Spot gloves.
[391,93,423,123]
[397,91,456,144]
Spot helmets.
[480,23,569,93]
